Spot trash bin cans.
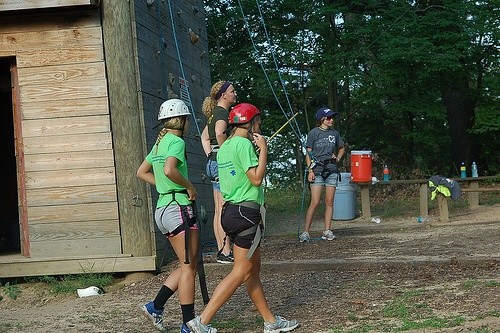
[332,172,355,220]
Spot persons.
[186,103,298,333]
[299,107,345,243]
[137,98,200,333]
[200,80,238,263]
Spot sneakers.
[321,230,336,241]
[217,252,234,263]
[186,316,217,333]
[141,301,167,333]
[263,315,298,333]
[180,324,190,333]
[299,231,310,243]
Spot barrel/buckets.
[351,151,373,183]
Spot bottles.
[471,161,478,179]
[411,216,429,225]
[383,165,389,181]
[461,162,466,179]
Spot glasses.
[323,115,335,121]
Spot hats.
[317,107,338,120]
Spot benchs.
[359,176,500,217]
[436,188,500,223]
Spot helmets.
[158,99,192,121]
[228,104,261,124]
